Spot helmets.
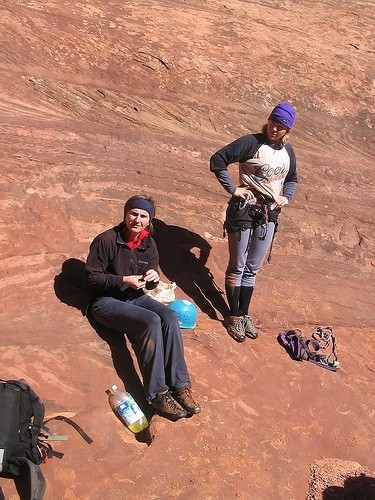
[168,299,198,329]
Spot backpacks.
[0,378,64,477]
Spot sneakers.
[224,314,246,342]
[173,388,202,414]
[243,315,258,339]
[151,389,187,421]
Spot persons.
[210,104,297,341]
[85,196,201,419]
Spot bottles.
[111,385,149,434]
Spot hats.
[267,104,295,129]
